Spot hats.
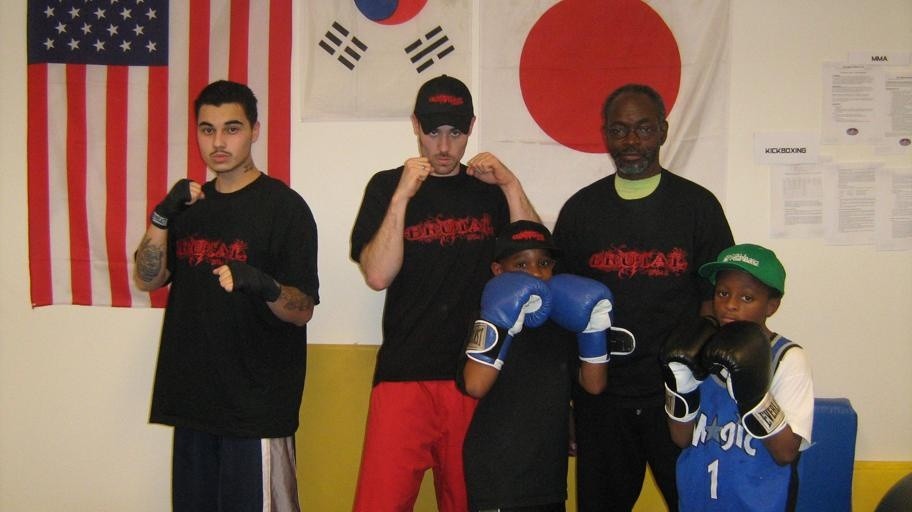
[416,74,474,135]
[699,245,786,294]
[491,219,556,261]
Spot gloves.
[467,271,552,369]
[546,273,636,366]
[658,320,716,424]
[702,320,786,439]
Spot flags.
[26,0,294,309]
[296,1,472,127]
[472,2,730,221]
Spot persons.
[454,221,612,512]
[349,76,547,510]
[659,242,815,508]
[135,80,320,511]
[550,81,737,509]
